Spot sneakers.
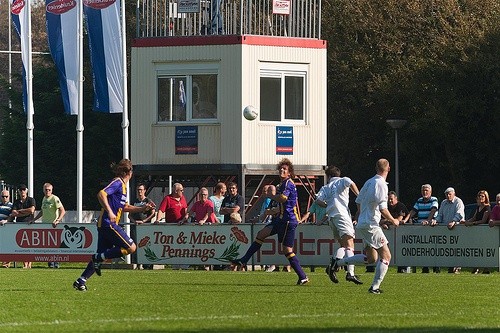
[92,252,104,276]
[368,286,381,295]
[325,264,339,283]
[346,272,363,285]
[71,278,88,293]
[296,276,309,286]
[229,259,246,268]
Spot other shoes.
[472,268,479,274]
[23,264,27,269]
[452,267,461,273]
[398,267,405,273]
[266,265,275,272]
[55,262,59,269]
[482,267,490,274]
[143,264,151,270]
[433,266,440,273]
[49,262,54,268]
[365,266,375,272]
[27,264,31,269]
[448,267,456,273]
[422,268,429,273]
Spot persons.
[154,183,192,270]
[330,159,399,294]
[430,186,465,274]
[0,189,15,268]
[230,158,311,286]
[488,193,500,227]
[400,184,441,273]
[460,190,492,274]
[248,185,276,273]
[11,183,37,268]
[297,193,329,226]
[315,165,364,285]
[209,182,227,271]
[380,191,412,273]
[219,181,245,271]
[227,212,245,272]
[73,159,157,292]
[177,187,215,271]
[128,183,156,270]
[27,183,66,268]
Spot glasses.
[200,193,207,197]
[19,188,26,191]
[479,195,484,197]
[2,196,8,198]
[177,190,183,192]
[46,189,51,191]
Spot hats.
[444,187,455,193]
[17,184,26,188]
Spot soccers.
[243,106,258,120]
